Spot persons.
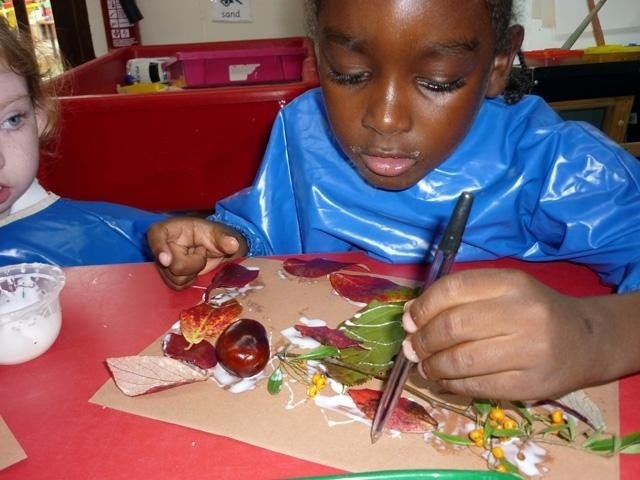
[0,15,177,268]
[148,0,640,402]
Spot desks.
[0,251,640,479]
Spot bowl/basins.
[0,263,65,367]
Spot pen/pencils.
[370,192,475,445]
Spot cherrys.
[214,319,270,373]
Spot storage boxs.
[175,46,307,87]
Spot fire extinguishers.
[100,0,143,53]
